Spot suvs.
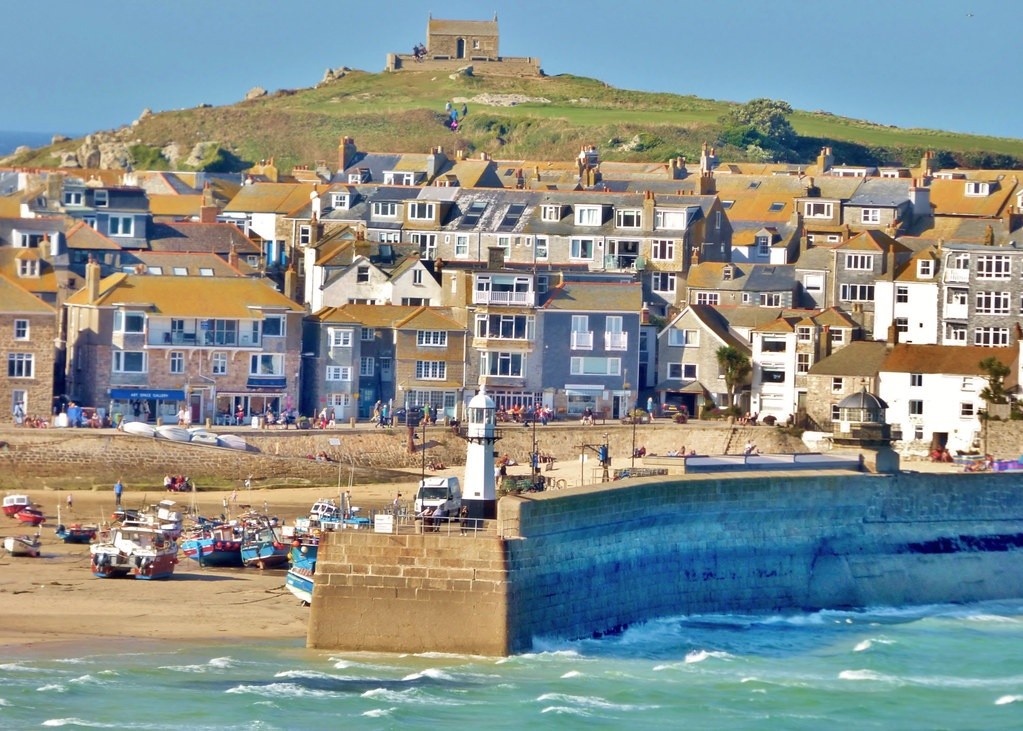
[378,405,437,427]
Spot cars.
[621,411,651,424]
[661,400,691,418]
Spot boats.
[156,425,191,443]
[88,456,377,604]
[191,431,219,446]
[1,532,41,558]
[54,524,96,543]
[217,433,249,450]
[1,494,32,517]
[13,505,46,526]
[122,421,155,437]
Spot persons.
[371,399,388,428]
[424,402,430,426]
[393,494,403,510]
[647,397,655,421]
[582,406,594,425]
[163,473,184,493]
[266,403,298,430]
[66,494,73,509]
[82,411,101,429]
[786,413,794,428]
[133,398,151,425]
[497,402,554,422]
[494,452,508,475]
[422,506,468,536]
[599,444,607,468]
[741,411,759,424]
[114,479,122,506]
[177,405,191,429]
[14,402,43,428]
[634,446,646,458]
[236,404,243,423]
[931,445,994,472]
[321,407,336,431]
[445,100,467,129]
[412,44,427,63]
[744,439,753,457]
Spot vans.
[413,476,462,522]
